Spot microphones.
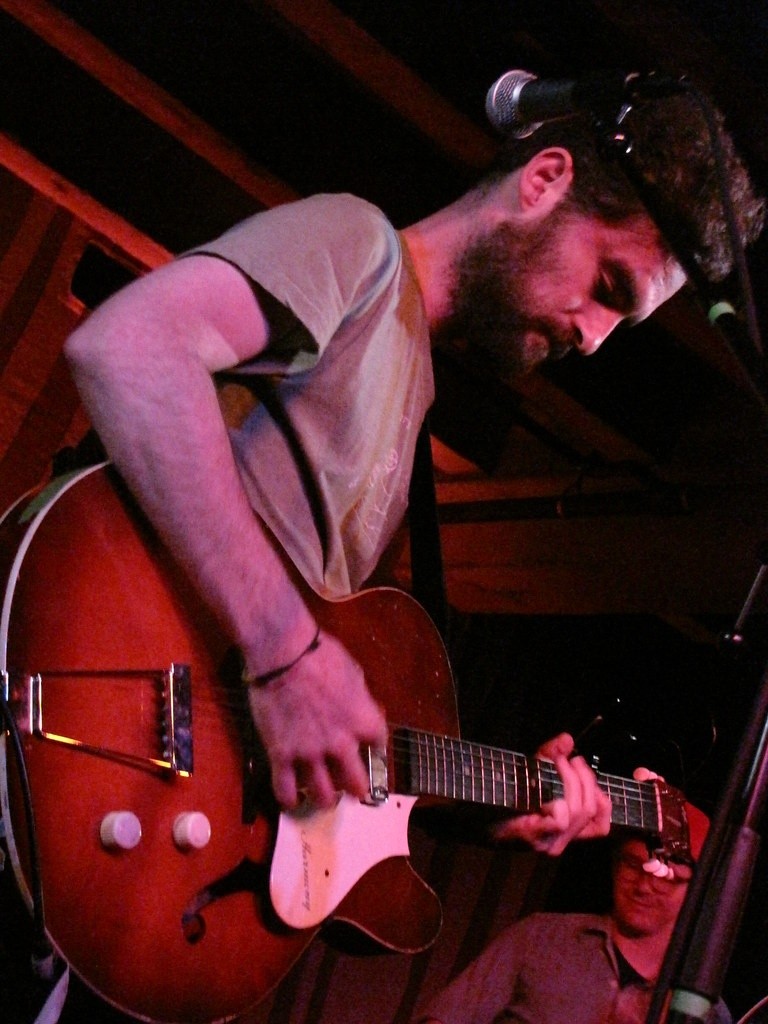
[485,69,687,138]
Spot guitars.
[1,443,708,1024]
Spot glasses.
[618,855,691,891]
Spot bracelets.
[238,620,330,691]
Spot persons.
[57,65,764,1024]
